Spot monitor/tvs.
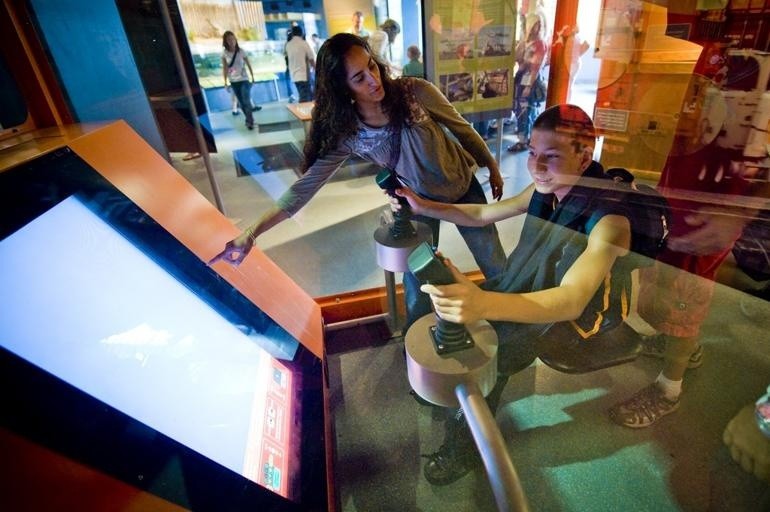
[0,143,330,511]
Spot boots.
[231,111,241,115]
[246,118,254,129]
[252,107,264,112]
[480,119,515,140]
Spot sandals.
[508,143,529,152]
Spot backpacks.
[533,80,548,102]
[625,168,673,262]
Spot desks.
[198,71,280,101]
[287,102,315,143]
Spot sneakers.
[642,332,705,366]
[609,383,682,429]
[423,438,484,486]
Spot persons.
[221,32,256,130]
[608,44,770,430]
[346,11,371,46]
[207,34,508,288]
[391,105,632,487]
[283,20,316,104]
[402,46,424,79]
[722,383,770,482]
[232,86,262,115]
[366,19,401,75]
[507,14,548,153]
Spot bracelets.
[754,388,770,433]
[245,229,257,246]
